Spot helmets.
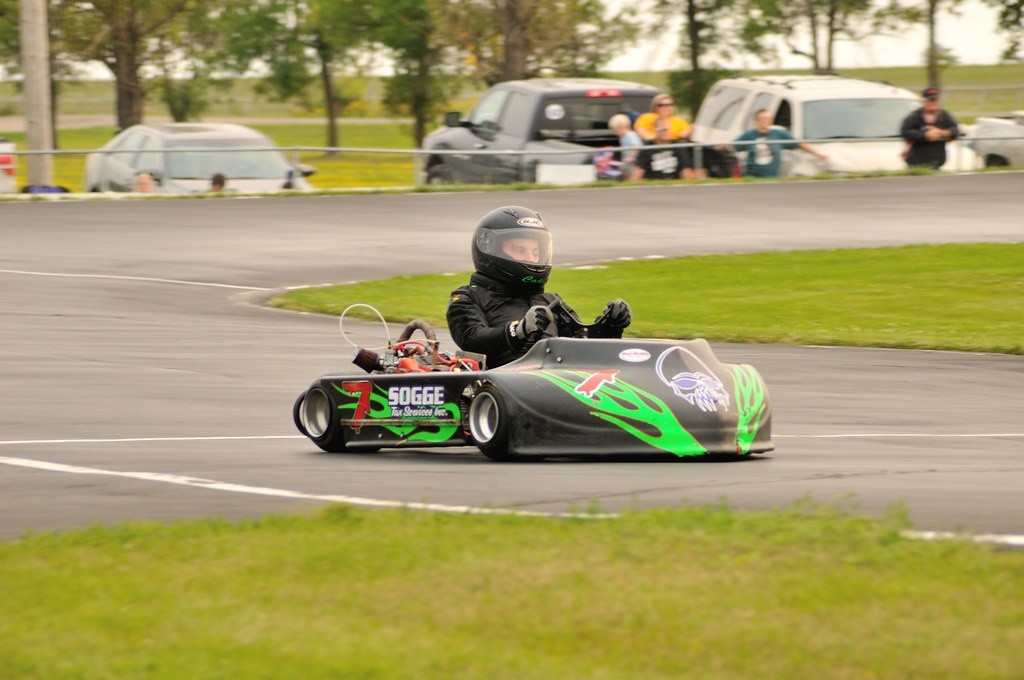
[472,206,551,291]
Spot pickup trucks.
[972,109,1024,173]
[417,78,741,185]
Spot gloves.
[603,298,632,328]
[513,305,558,342]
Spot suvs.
[686,72,987,176]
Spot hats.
[923,88,941,100]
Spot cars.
[0,138,19,195]
[82,120,318,194]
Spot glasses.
[656,102,673,107]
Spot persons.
[713,108,831,178]
[900,87,959,171]
[608,93,695,180]
[446,205,631,371]
